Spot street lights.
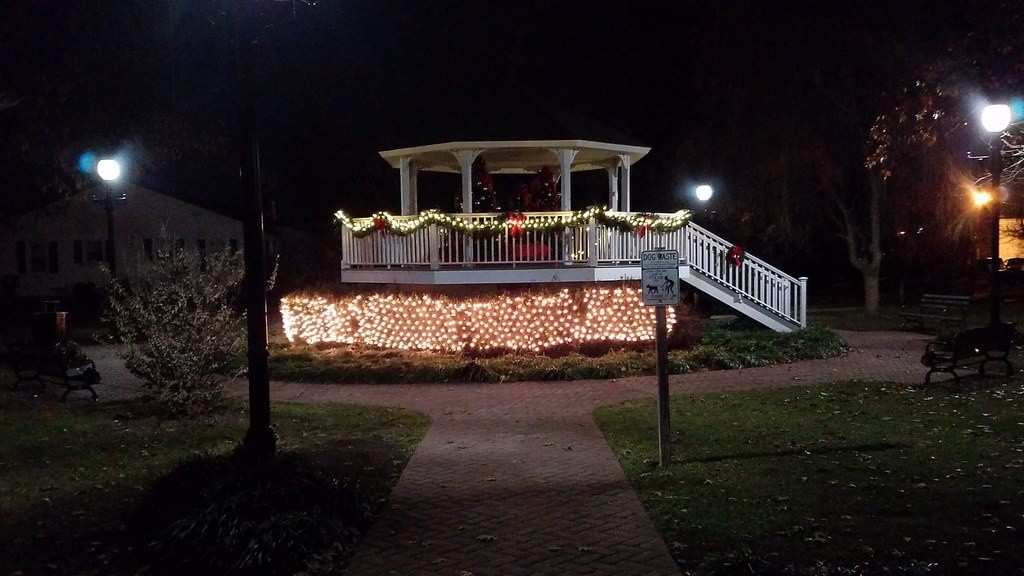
[92,157,127,285]
[967,103,1011,329]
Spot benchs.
[0,337,101,403]
[921,319,1016,386]
[899,294,972,333]
